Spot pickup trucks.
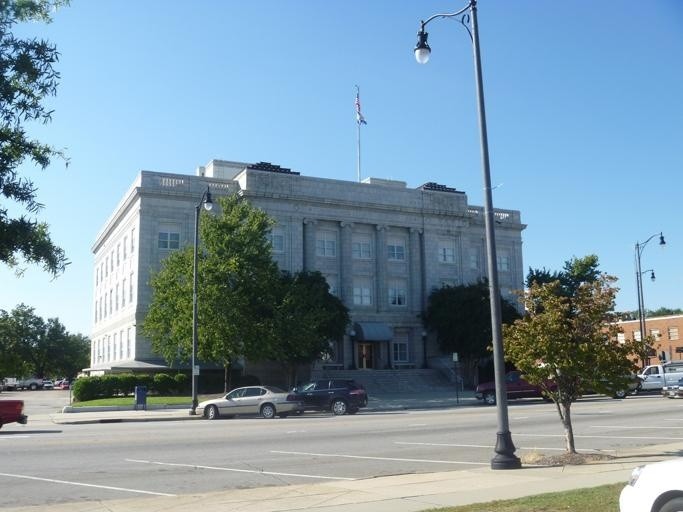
[630,365,683,396]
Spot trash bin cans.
[135,385,147,410]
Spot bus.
[661,376,683,400]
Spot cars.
[0,400,27,428]
[476,363,640,405]
[619,458,683,512]
[195,379,368,420]
[0,378,69,390]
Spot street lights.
[453,352,459,405]
[189,186,213,415]
[413,0,521,470]
[634,232,666,364]
